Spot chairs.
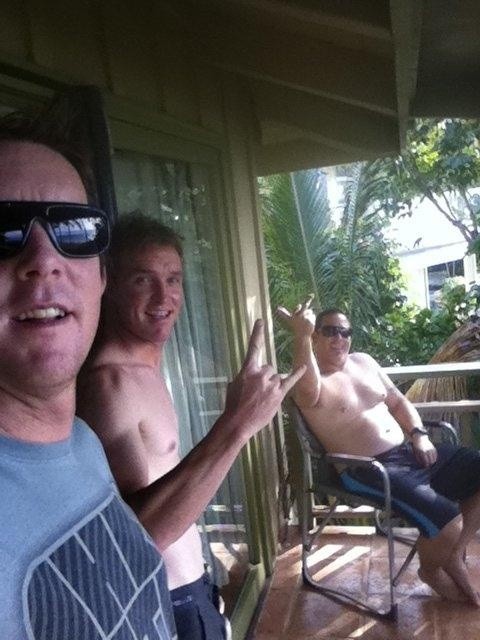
[283,398,464,621]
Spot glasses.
[322,325,351,337]
[0,200,110,259]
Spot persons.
[76,213,307,640]
[276,294,480,608]
[0,112,178,640]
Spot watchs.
[410,427,428,438]
